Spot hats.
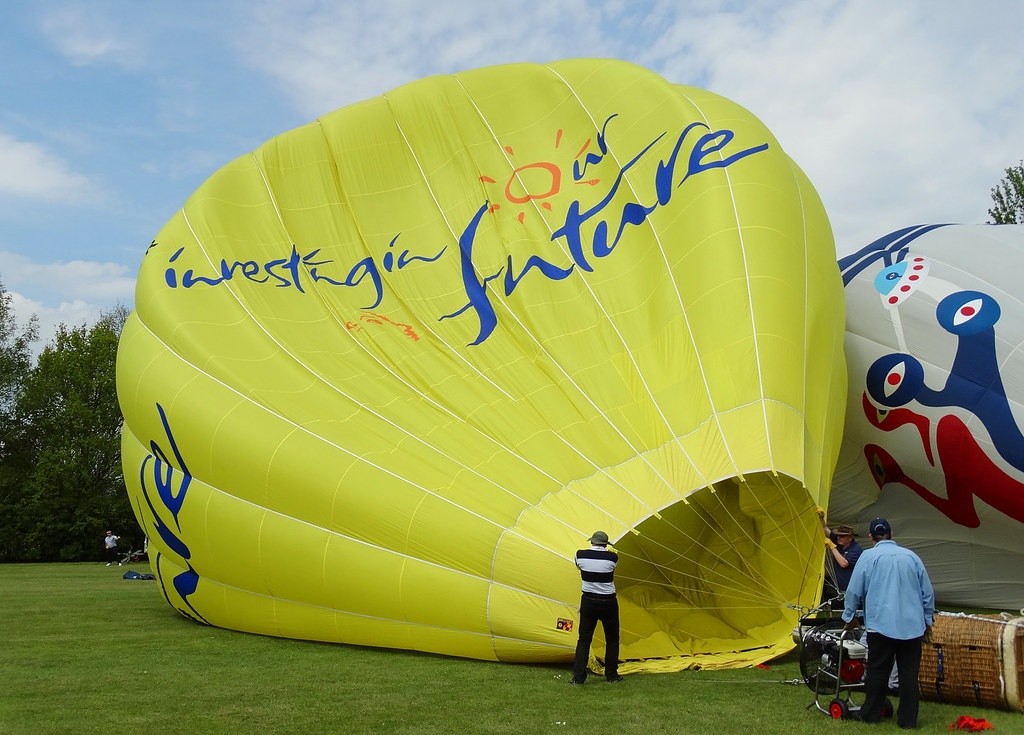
[870,518,891,535]
[833,525,860,537]
[587,531,608,544]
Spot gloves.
[824,538,837,550]
[816,505,825,518]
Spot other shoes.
[106,563,111,567]
[568,679,583,684]
[608,676,624,682]
[847,709,882,722]
[119,562,123,566]
[897,718,911,729]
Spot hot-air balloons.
[114,52,1023,713]
[822,221,1023,612]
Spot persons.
[840,518,934,730]
[814,505,864,592]
[105,530,122,567]
[567,532,624,685]
